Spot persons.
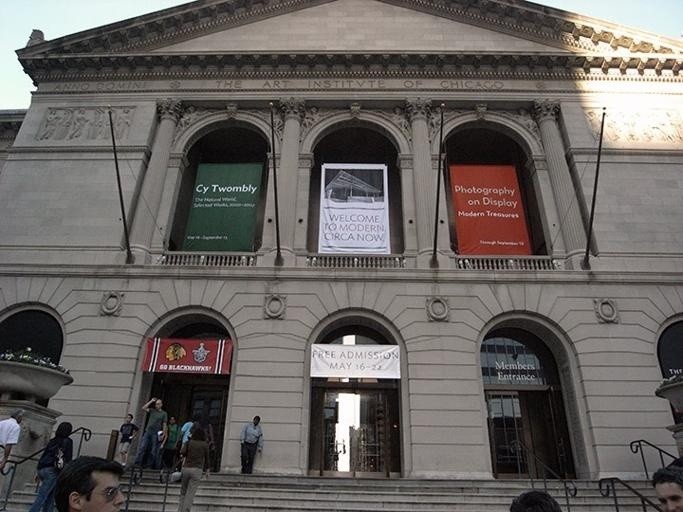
[190,414,216,471]
[161,415,179,470]
[237,415,262,474]
[178,414,198,468]
[27,421,73,512]
[651,464,682,512]
[507,488,561,512]
[54,454,127,512]
[116,411,138,466]
[132,397,168,470]
[176,425,210,512]
[0,408,25,472]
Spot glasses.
[84,484,124,502]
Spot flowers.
[655,369,682,387]
[0,344,73,374]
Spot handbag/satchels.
[54,448,64,470]
